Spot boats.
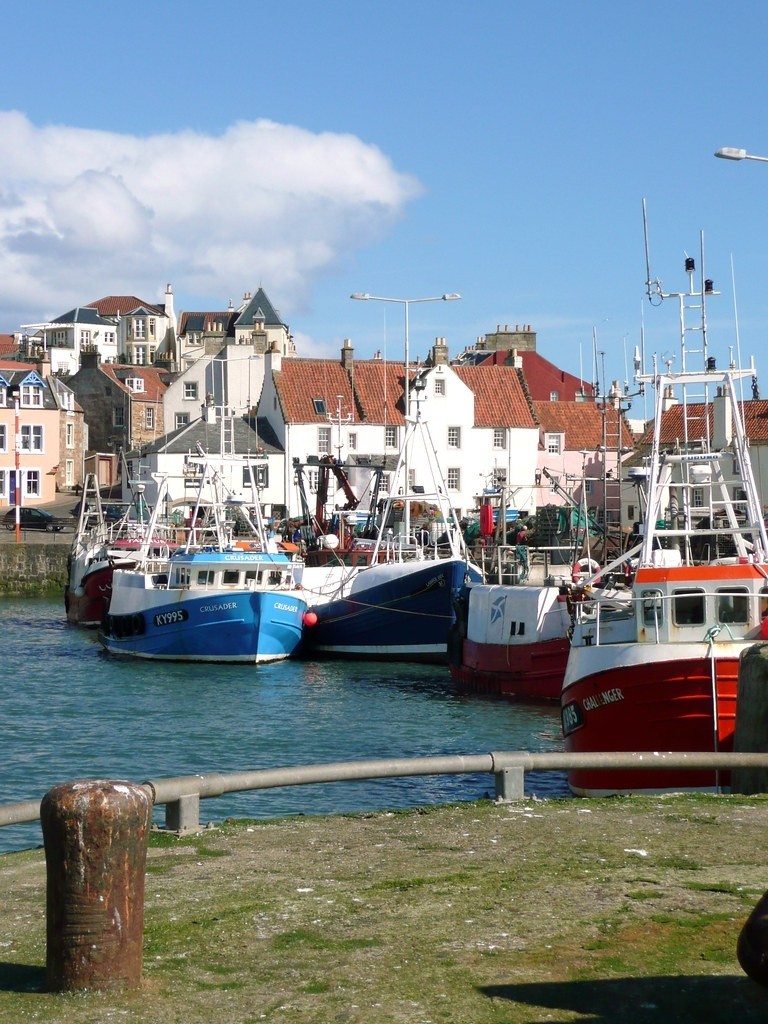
[300,357,492,664]
[63,444,179,630]
[448,323,644,709]
[561,197,768,796]
[100,447,307,664]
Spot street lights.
[180,355,264,498]
[348,292,461,545]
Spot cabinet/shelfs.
[310,549,394,567]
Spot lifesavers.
[572,557,601,587]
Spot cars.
[3,507,64,533]
